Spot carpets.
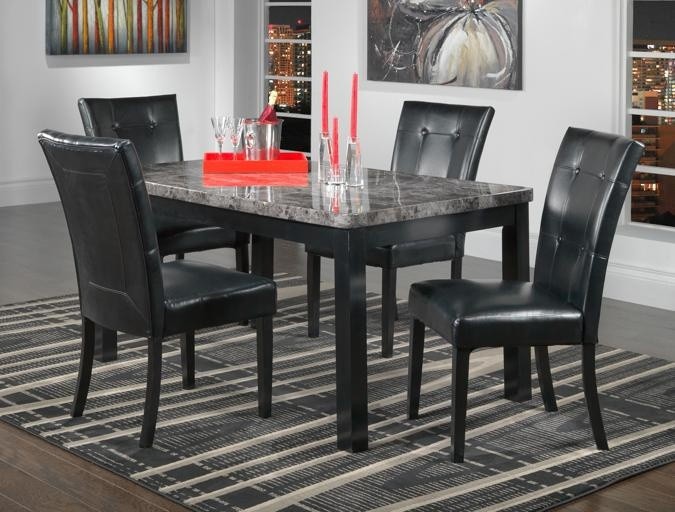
[0,264,674,512]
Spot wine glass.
[211,115,246,162]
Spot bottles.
[257,90,279,123]
[317,131,367,188]
[320,180,370,223]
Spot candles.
[321,70,359,175]
